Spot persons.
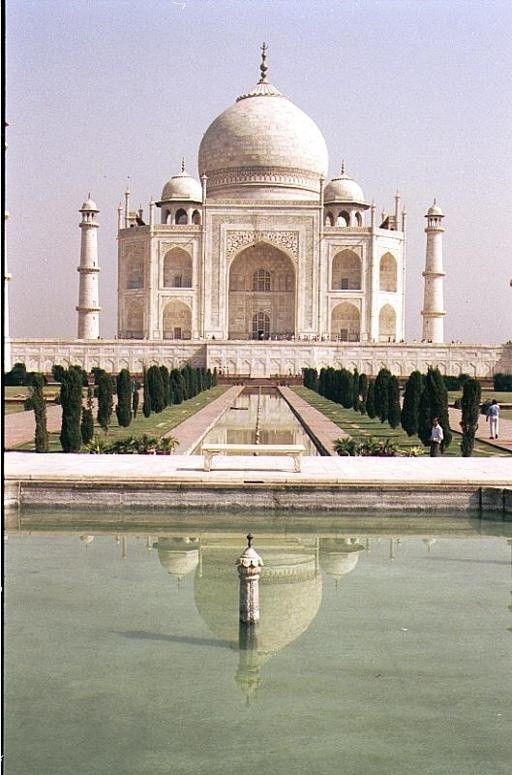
[486,399,501,439]
[430,416,444,457]
